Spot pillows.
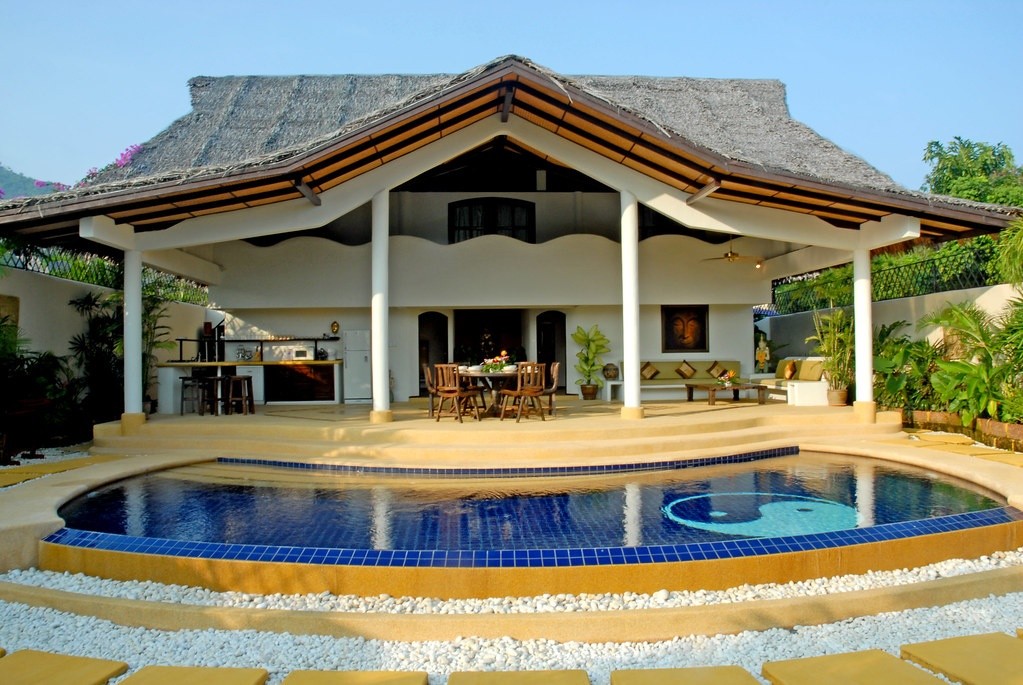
[706,360,728,380]
[784,359,797,380]
[674,360,696,380]
[641,361,660,380]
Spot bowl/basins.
[467,365,482,372]
[501,364,517,372]
[456,366,467,372]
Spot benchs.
[619,361,751,402]
[748,357,834,405]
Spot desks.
[453,370,538,419]
[154,360,344,415]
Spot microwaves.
[292,346,315,360]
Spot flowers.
[480,350,509,373]
[718,370,736,386]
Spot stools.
[203,376,230,417]
[179,376,201,416]
[228,374,254,416]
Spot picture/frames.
[660,305,710,353]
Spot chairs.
[422,362,560,423]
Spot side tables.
[601,381,623,403]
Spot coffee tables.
[684,383,768,405]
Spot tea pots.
[234,345,246,359]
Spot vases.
[601,363,619,381]
[725,383,732,387]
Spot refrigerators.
[342,330,372,399]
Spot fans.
[702,234,764,262]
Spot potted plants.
[570,324,611,400]
[106,278,180,420]
[805,298,855,407]
